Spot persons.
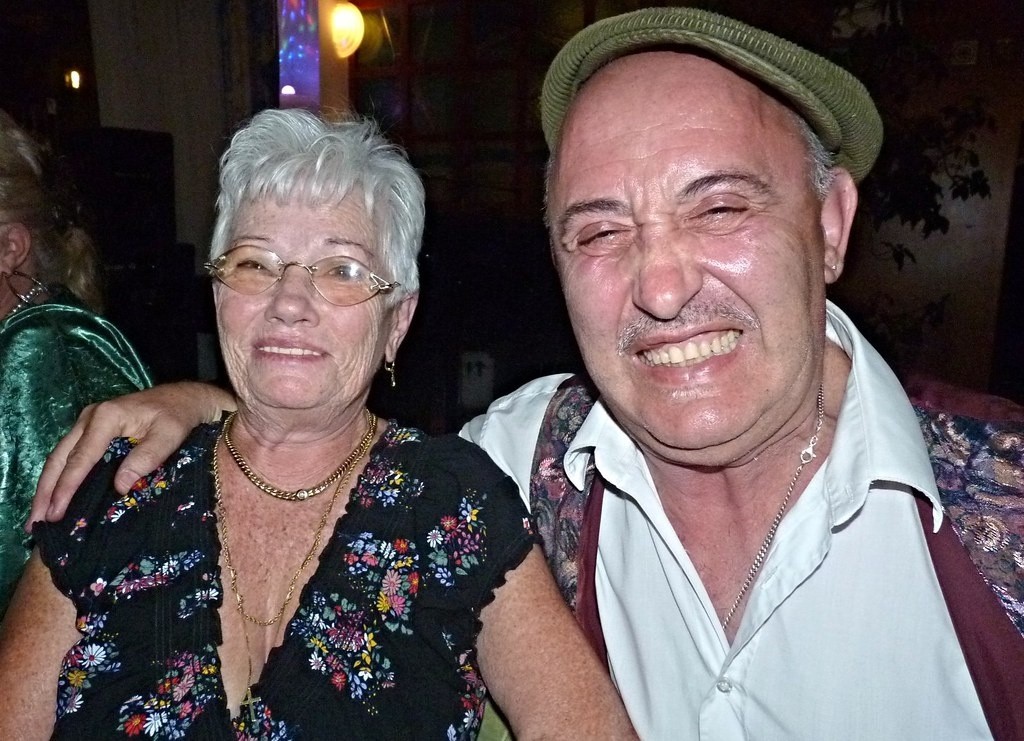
[223,404,376,502]
[23,7,1024,741]
[211,411,380,733]
[0,106,645,740]
[1,111,162,624]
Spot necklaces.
[715,376,826,636]
[0,274,51,324]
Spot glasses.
[203,245,399,305]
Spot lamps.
[329,1,394,69]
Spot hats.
[541,8,884,184]
